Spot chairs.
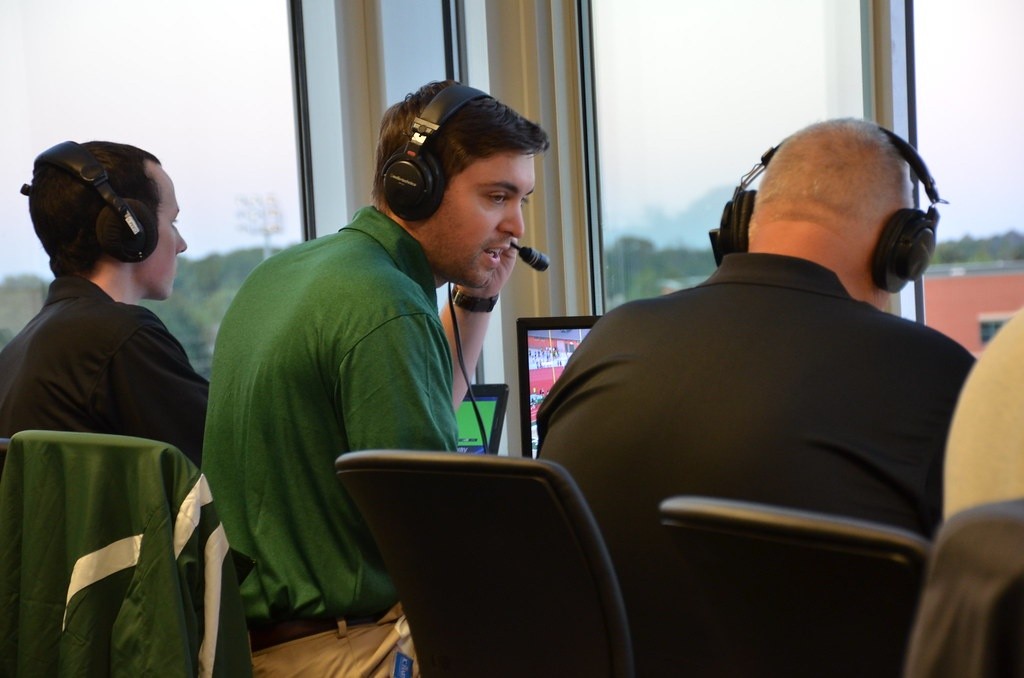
[0,431,254,678]
[648,499,926,678]
[908,497,1024,678]
[335,450,632,678]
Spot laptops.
[455,384,510,456]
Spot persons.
[202,78,549,678]
[943,304,1024,678]
[537,117,978,678]
[0,140,209,466]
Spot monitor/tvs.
[515,315,600,460]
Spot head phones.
[31,141,158,263]
[708,127,950,292]
[378,86,493,222]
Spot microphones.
[509,243,550,271]
